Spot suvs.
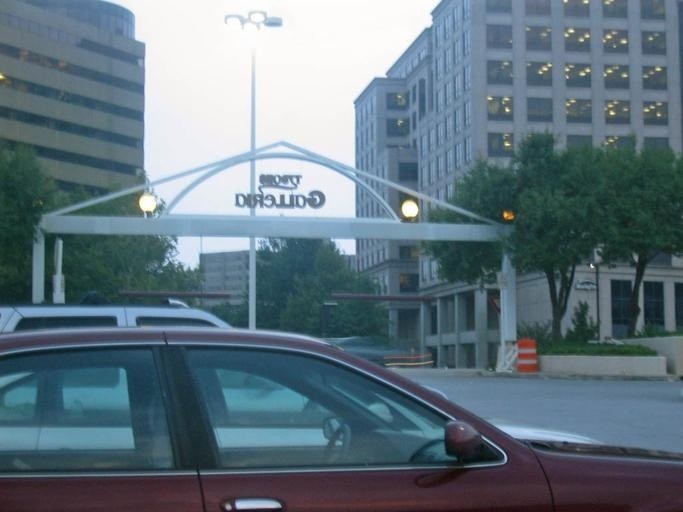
[0,294,327,415]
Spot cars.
[0,326,682,512]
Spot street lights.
[220,8,284,333]
